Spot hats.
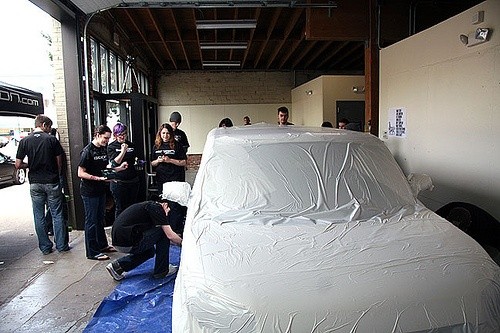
[170,112,181,122]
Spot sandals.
[102,245,116,252]
[89,253,110,261]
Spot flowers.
[134,157,147,167]
[100,162,117,176]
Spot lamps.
[203,61,240,66]
[459,27,490,47]
[199,43,248,49]
[195,19,256,28]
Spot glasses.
[117,133,126,138]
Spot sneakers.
[106,263,124,280]
[166,267,177,277]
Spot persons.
[77,125,117,261]
[243,116,251,125]
[150,111,190,196]
[321,118,361,132]
[219,118,233,127]
[106,199,182,281]
[277,107,294,125]
[15,115,70,254]
[107,123,142,219]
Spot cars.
[164,126,500,333]
[20,132,31,140]
[0,136,9,148]
[0,152,29,186]
